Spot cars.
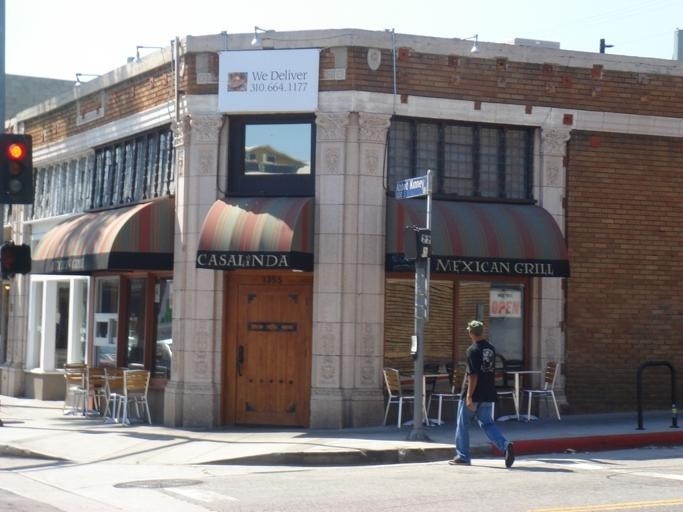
[80,312,171,371]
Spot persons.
[447,319,516,469]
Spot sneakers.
[448,459,471,465]
[504,443,515,467]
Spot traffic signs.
[394,178,430,199]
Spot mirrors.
[244,124,312,175]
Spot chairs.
[490,386,519,421]
[60,361,152,426]
[381,365,430,428]
[423,364,467,426]
[518,361,562,422]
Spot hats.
[465,319,483,333]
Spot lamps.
[252,26,268,46]
[74,73,99,87]
[462,35,478,54]
[133,45,163,63]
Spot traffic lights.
[0,244,29,275]
[0,132,32,204]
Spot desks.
[396,369,454,425]
[495,369,546,422]
